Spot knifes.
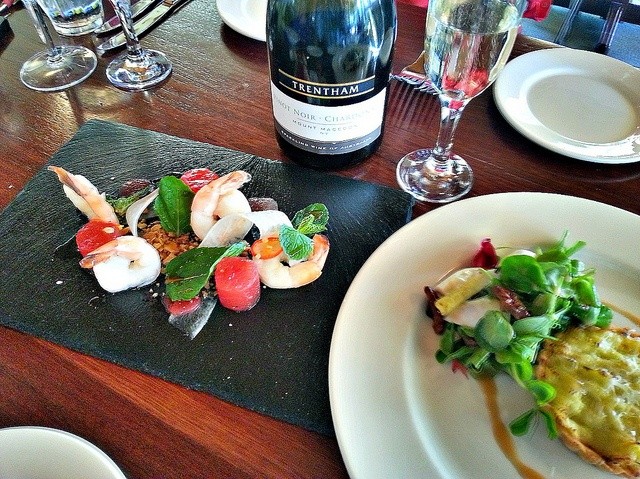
[95,0,155,33]
[97,0,190,50]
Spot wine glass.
[20,1,97,92]
[396,1,518,204]
[106,0,172,91]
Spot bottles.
[266,0,398,171]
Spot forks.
[415,78,439,95]
[391,50,426,86]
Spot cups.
[37,0,104,37]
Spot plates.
[0,425,128,479]
[216,0,267,42]
[492,48,640,164]
[327,192,639,478]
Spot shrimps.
[189,169,252,243]
[78,235,162,294]
[47,165,120,228]
[251,232,331,290]
